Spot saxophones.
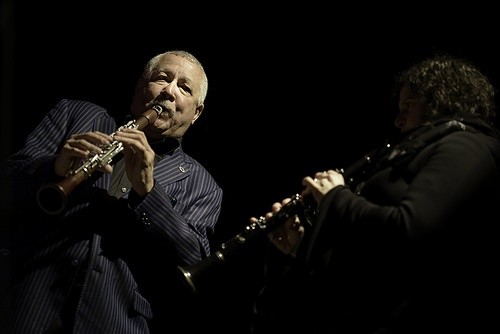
[36,105,162,215]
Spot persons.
[251,57,500,334]
[0,51,223,333]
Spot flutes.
[176,130,410,293]
